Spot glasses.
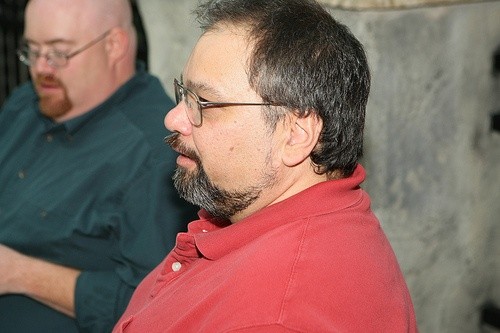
[16,29,112,68]
[173,78,288,128]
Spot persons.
[111,1,418,333]
[0,0,200,333]
